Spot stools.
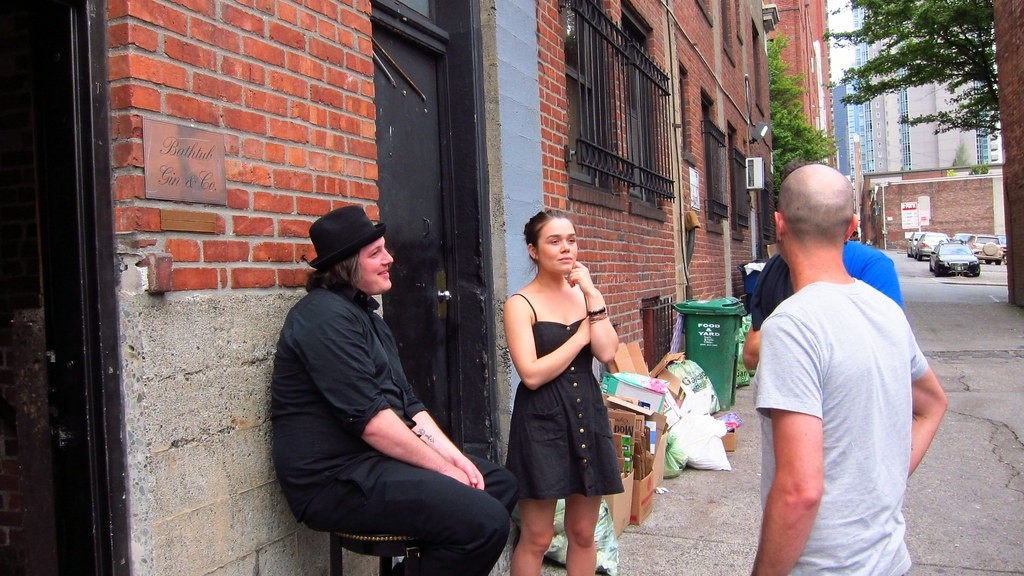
[329,532,421,576]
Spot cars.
[907,232,927,259]
[929,242,981,277]
[914,232,952,262]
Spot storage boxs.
[721,429,737,452]
[601,341,686,540]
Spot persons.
[502,209,619,576]
[742,160,948,576]
[271,206,522,576]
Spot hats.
[308,205,387,270]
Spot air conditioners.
[745,157,765,189]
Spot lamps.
[749,122,769,145]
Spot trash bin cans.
[671,296,748,411]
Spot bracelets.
[586,307,611,325]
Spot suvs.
[952,233,977,245]
[963,234,1003,266]
[995,234,1008,265]
